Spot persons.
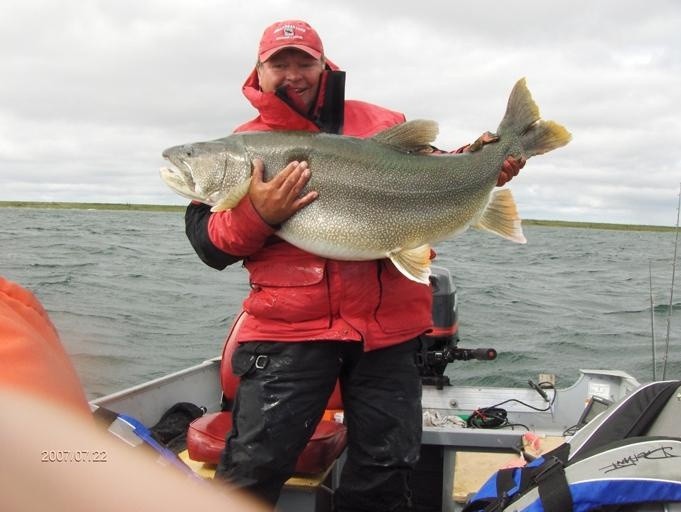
[2,280,276,510]
[182,17,530,512]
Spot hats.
[258,20,323,62]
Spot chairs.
[187,307,348,473]
